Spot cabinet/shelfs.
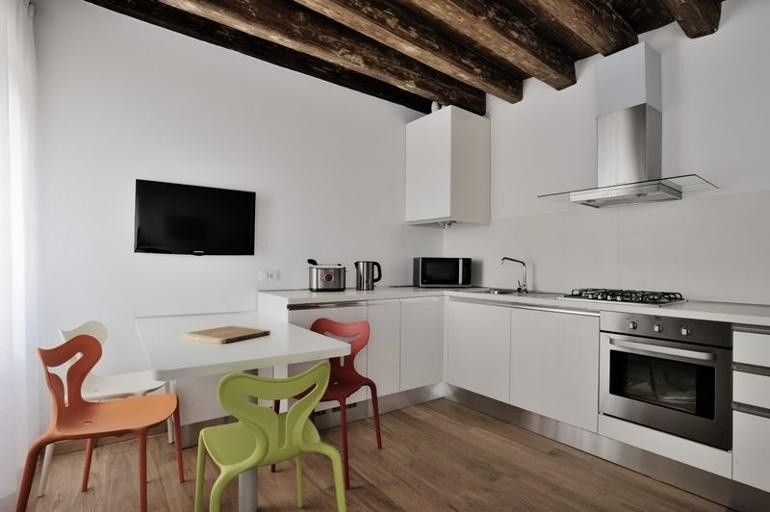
[510,309,600,458]
[259,298,444,431]
[732,331,770,512]
[406,105,491,224]
[444,301,512,421]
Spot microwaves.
[413,257,474,289]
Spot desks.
[134,310,352,512]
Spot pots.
[306,259,346,292]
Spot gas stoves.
[556,286,686,306]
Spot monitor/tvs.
[134,179,256,256]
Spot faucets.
[500,257,527,292]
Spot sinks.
[454,288,513,295]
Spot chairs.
[194,361,347,512]
[38,321,173,498]
[16,333,184,512]
[271,317,381,489]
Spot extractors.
[538,104,720,210]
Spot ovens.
[598,310,733,451]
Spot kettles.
[354,260,381,290]
